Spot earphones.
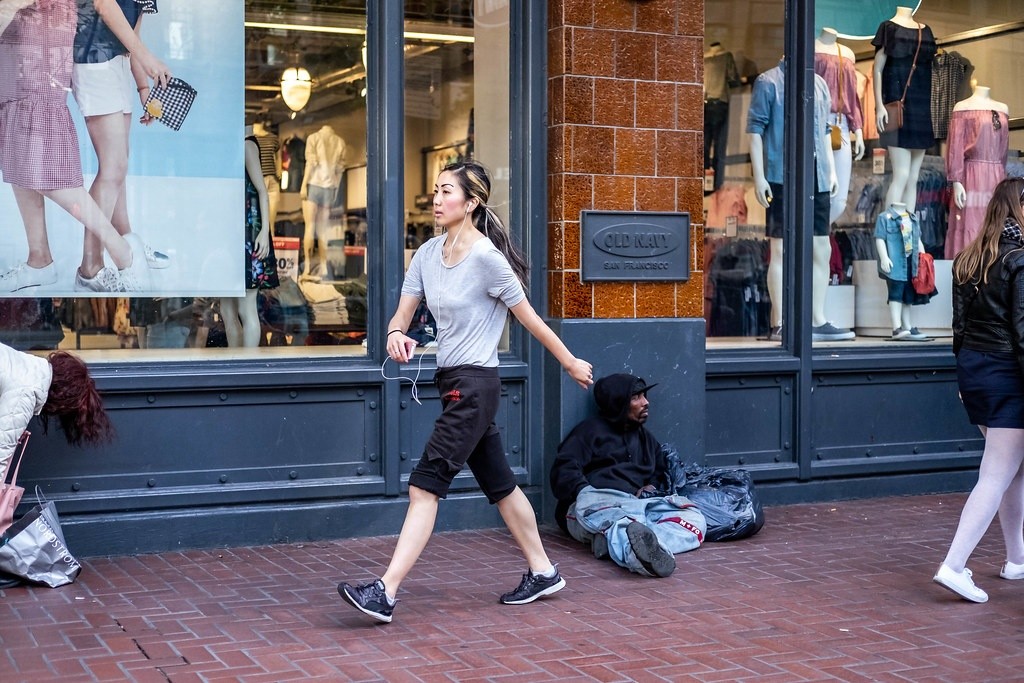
[467,202,472,212]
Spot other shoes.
[901,327,926,339]
[811,321,856,340]
[590,534,608,558]
[626,522,676,577]
[892,328,911,340]
[770,327,783,341]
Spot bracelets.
[386,330,403,337]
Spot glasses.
[992,109,1001,130]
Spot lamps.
[286,111,297,120]
[279,47,313,111]
[353,80,366,98]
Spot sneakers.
[74,265,131,292]
[117,233,154,292]
[933,562,989,603]
[0,260,58,292]
[499,562,566,605]
[337,578,401,623]
[999,559,1024,580]
[142,244,172,269]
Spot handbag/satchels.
[143,77,198,131]
[830,125,842,150]
[0,485,82,589]
[876,100,903,134]
[0,430,29,536]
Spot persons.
[300,126,346,276]
[704,42,742,190]
[278,132,307,192]
[944,85,1013,259]
[338,159,593,623]
[219,131,278,350]
[0,0,172,293]
[874,202,929,341]
[126,296,159,350]
[872,5,939,215]
[0,339,114,476]
[813,27,866,225]
[743,54,856,342]
[927,177,1024,605]
[254,122,281,240]
[550,374,706,578]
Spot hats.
[631,380,660,396]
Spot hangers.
[702,222,768,242]
[830,221,876,235]
[850,150,1024,185]
[723,175,753,189]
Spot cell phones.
[405,343,416,359]
